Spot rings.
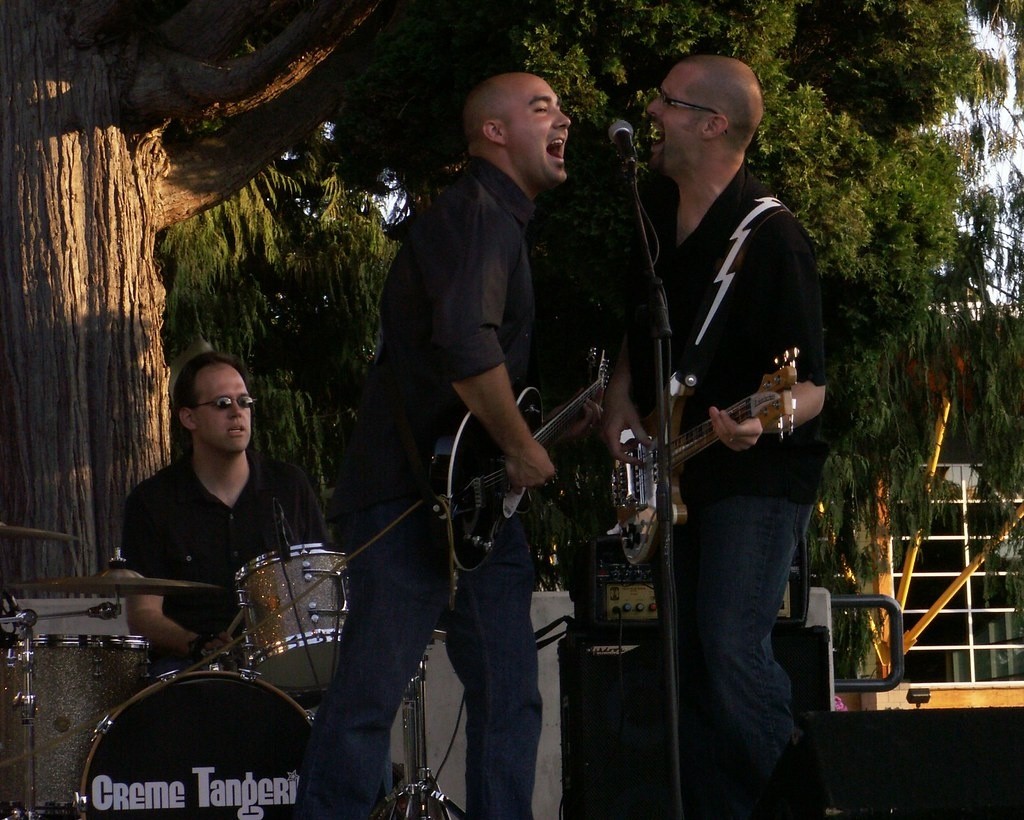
[730,438,734,442]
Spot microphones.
[274,499,291,563]
[609,120,636,170]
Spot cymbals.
[10,568,223,594]
[0,520,82,543]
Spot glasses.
[189,394,257,410]
[654,84,727,136]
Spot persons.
[291,73,604,820]
[125,354,331,683]
[602,56,825,820]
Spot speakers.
[553,621,1024,820]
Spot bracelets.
[191,632,212,662]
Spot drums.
[78,670,313,820]
[234,540,350,688]
[0,634,149,819]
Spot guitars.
[611,346,800,572]
[431,344,611,570]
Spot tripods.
[371,659,466,820]
